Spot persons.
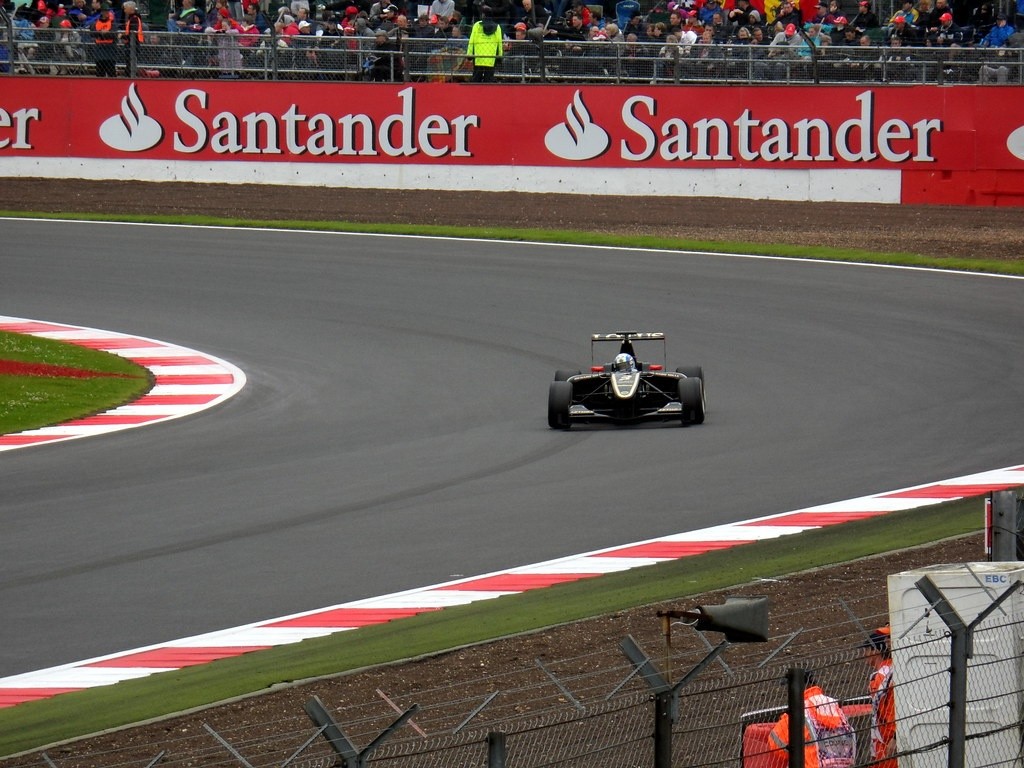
[89,4,120,78]
[145,32,173,79]
[766,670,857,768]
[861,628,900,768]
[166,0,468,84]
[614,353,637,373]
[876,0,1024,84]
[468,5,504,84]
[505,0,625,81]
[118,1,145,79]
[626,0,875,80]
[0,0,115,76]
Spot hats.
[0,0,1012,40]
[781,672,815,684]
[856,634,886,649]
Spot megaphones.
[672,597,769,644]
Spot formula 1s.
[547,330,707,430]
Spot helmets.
[615,353,635,371]
